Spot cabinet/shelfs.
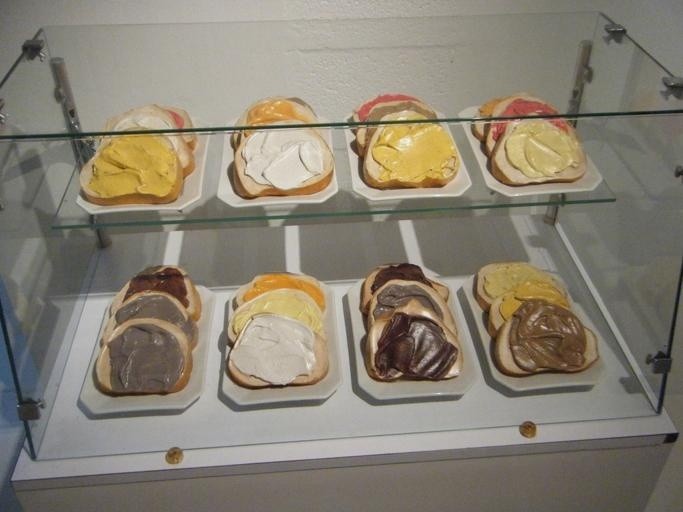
[2,11,682,512]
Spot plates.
[463,271,607,391]
[343,112,470,199]
[459,103,603,195]
[78,282,216,416]
[219,278,342,407]
[74,114,211,215]
[216,117,338,210]
[347,274,478,403]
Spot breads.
[474,261,600,377]
[79,103,198,205]
[348,92,460,191]
[225,271,330,390]
[469,94,587,187]
[91,264,203,397]
[229,96,337,202]
[359,264,465,383]
